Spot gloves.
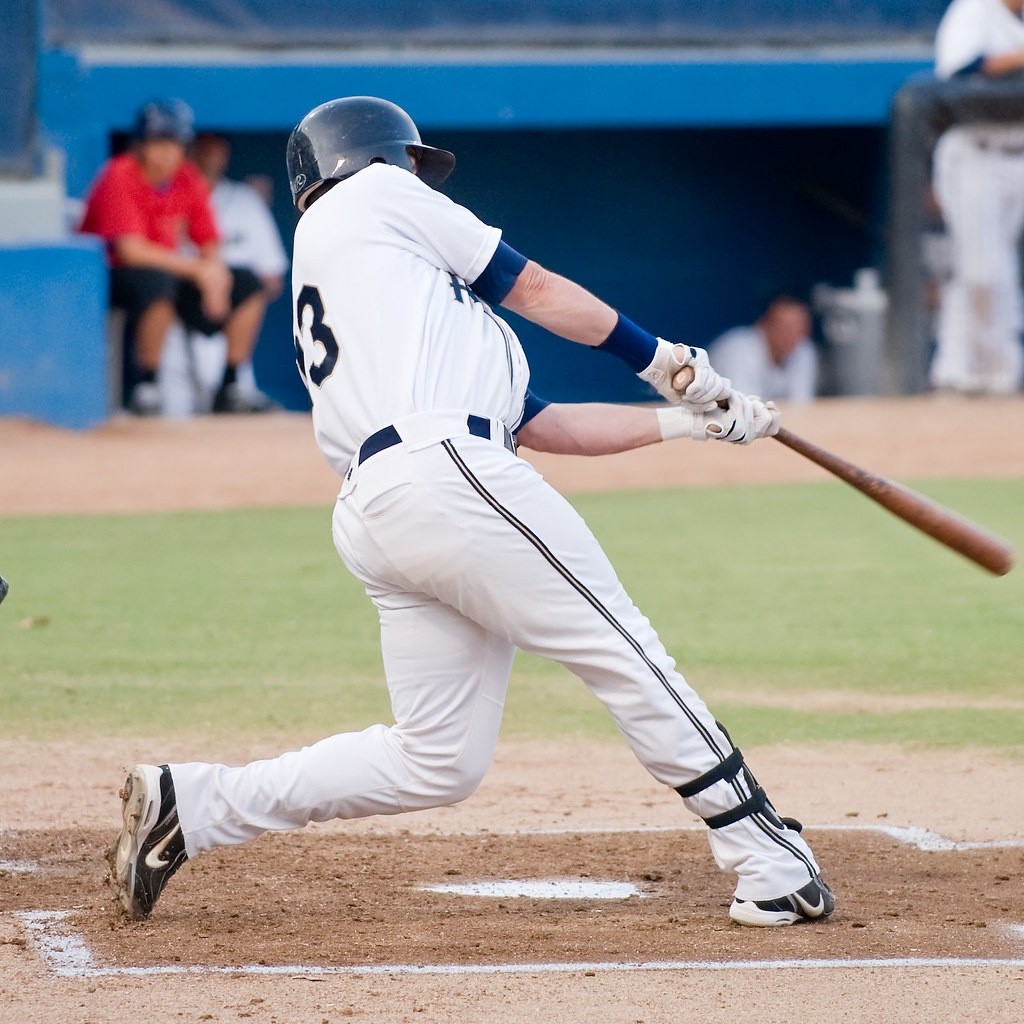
[636,335,734,412]
[657,386,781,445]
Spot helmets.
[136,96,196,144]
[287,97,456,211]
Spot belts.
[358,413,516,465]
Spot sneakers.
[727,871,835,929]
[104,763,190,921]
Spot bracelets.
[657,406,694,440]
[594,308,659,374]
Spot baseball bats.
[668,363,1016,579]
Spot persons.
[112,98,841,928]
[83,89,290,417]
[700,0,1024,395]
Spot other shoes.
[212,390,269,414]
